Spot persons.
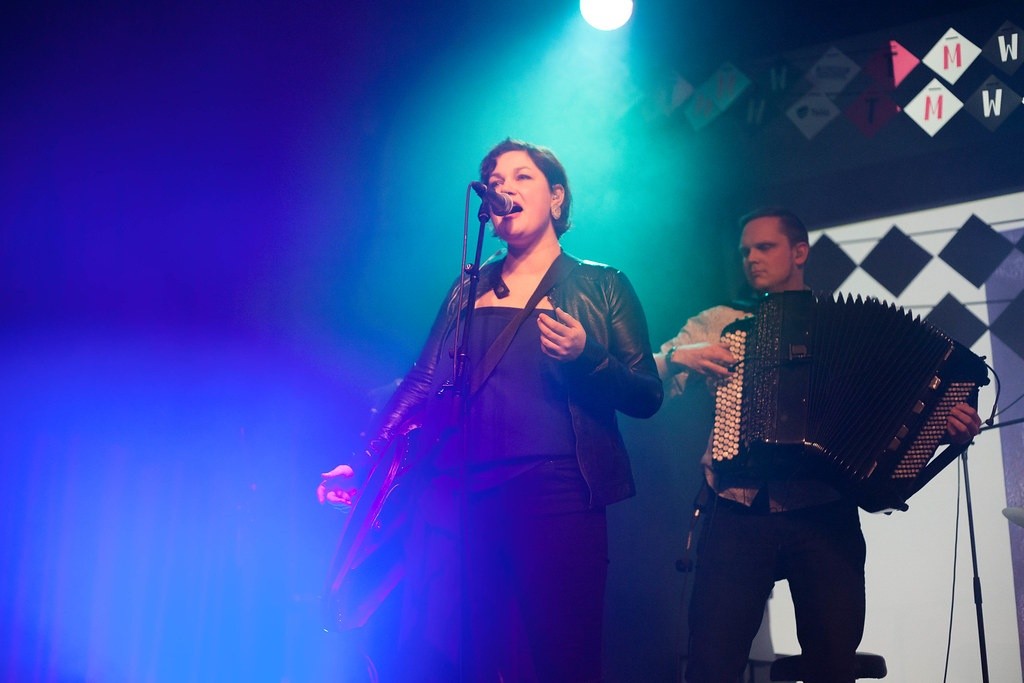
[648,204,982,683]
[313,137,666,683]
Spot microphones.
[471,181,513,216]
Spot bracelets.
[665,345,677,373]
